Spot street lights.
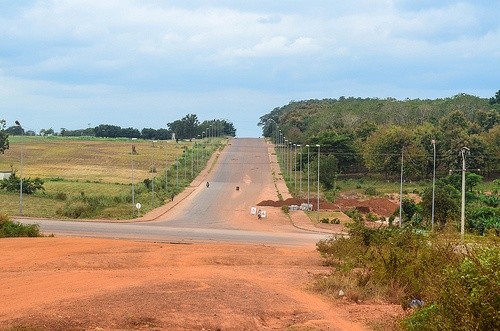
[132,118,232,218]
[431,139,436,233]
[15,120,24,214]
[266,119,321,224]
